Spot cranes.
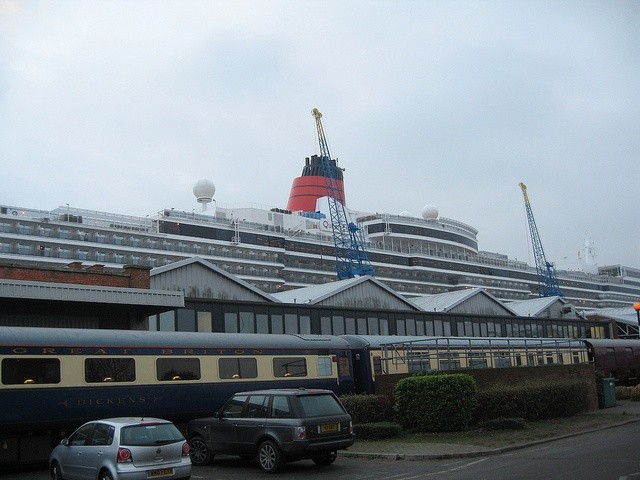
[519,182,567,297]
[310,108,377,280]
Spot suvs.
[186,387,356,473]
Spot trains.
[0,326,640,435]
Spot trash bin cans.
[599,377,616,408]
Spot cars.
[48,417,192,480]
